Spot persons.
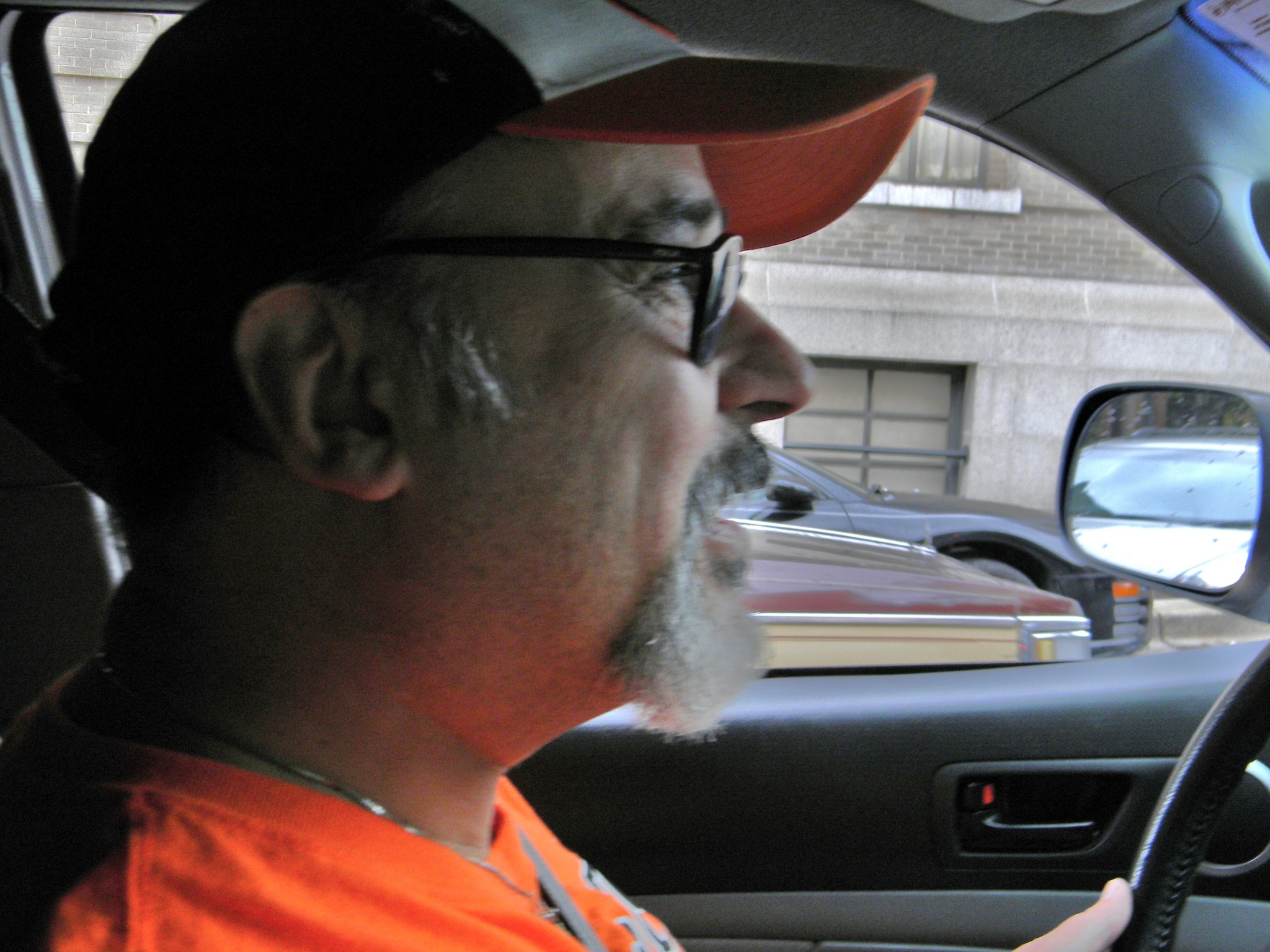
[0,0,1133,952]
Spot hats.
[52,1,935,507]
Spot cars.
[720,441,1153,670]
[1071,427,1261,589]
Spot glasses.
[334,230,747,368]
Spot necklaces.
[88,651,572,933]
